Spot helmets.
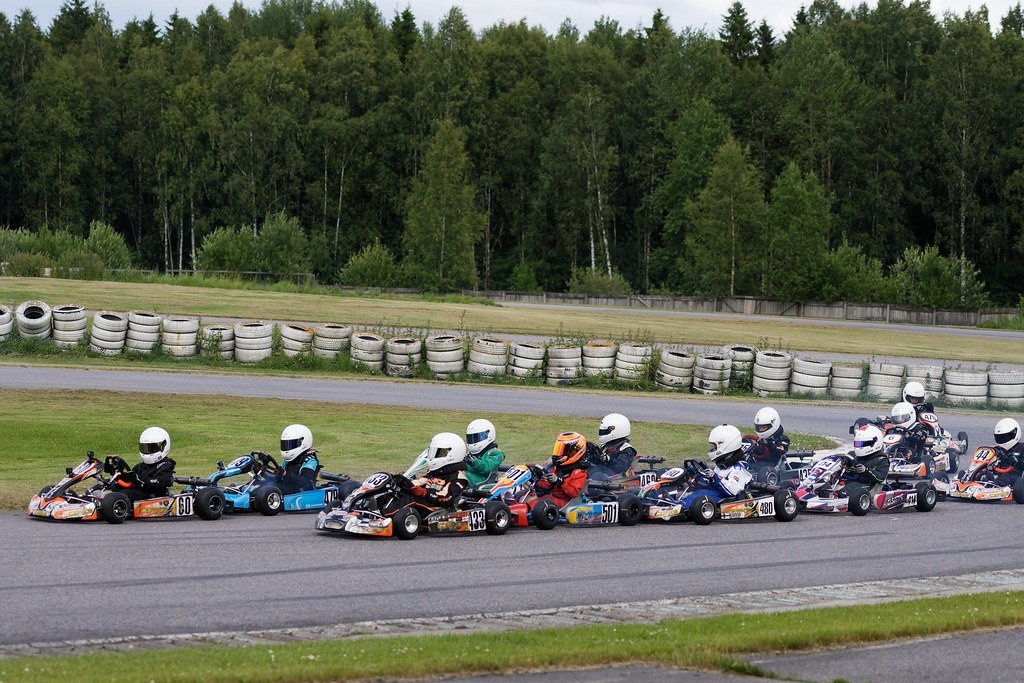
[994,418,1021,450]
[708,424,743,461]
[599,412,631,444]
[467,418,496,453]
[852,424,882,456]
[552,432,586,465]
[755,406,781,438]
[902,381,925,407]
[138,426,170,464]
[426,432,466,472]
[891,401,916,431]
[281,424,313,460]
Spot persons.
[816,425,891,498]
[462,419,506,491]
[586,413,637,481]
[649,424,753,511]
[372,432,469,515]
[898,382,944,436]
[229,424,324,495]
[527,431,589,513]
[957,417,1024,487]
[884,402,928,466]
[742,407,790,474]
[66,427,177,505]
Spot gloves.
[275,465,287,477]
[104,462,115,473]
[125,470,136,481]
[856,464,866,474]
[600,453,611,464]
[410,485,427,496]
[545,472,558,482]
[1004,453,1016,466]
[758,438,770,447]
[909,434,923,444]
[704,468,714,478]
